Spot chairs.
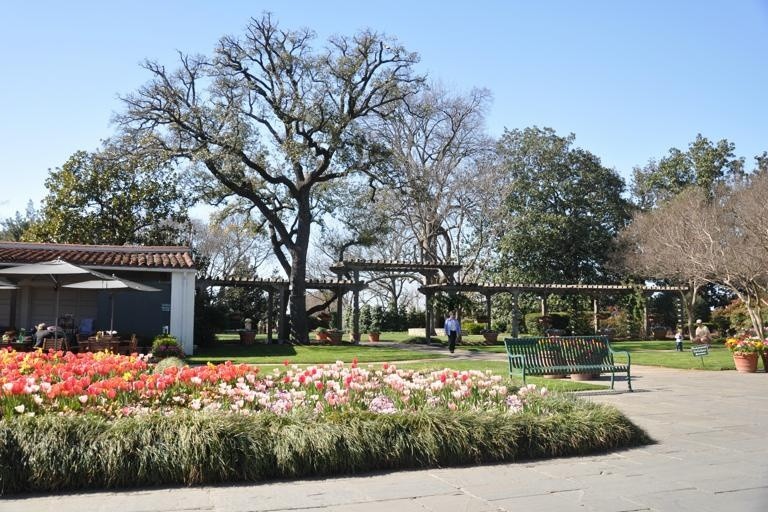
[37,334,138,354]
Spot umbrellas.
[0,256,163,354]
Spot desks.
[0,341,35,352]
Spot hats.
[696,319,702,323]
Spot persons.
[691,318,712,346]
[674,328,684,351]
[245,318,252,331]
[258,319,264,334]
[444,310,461,353]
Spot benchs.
[503,334,632,392]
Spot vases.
[603,333,614,341]
[734,352,758,373]
[485,333,496,345]
[541,356,602,380]
[761,353,768,372]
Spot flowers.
[599,328,615,334]
[726,332,762,352]
[759,340,768,352]
[479,329,498,337]
[538,336,608,360]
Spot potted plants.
[655,327,667,339]
[316,327,328,339]
[367,327,380,342]
[238,330,257,344]
